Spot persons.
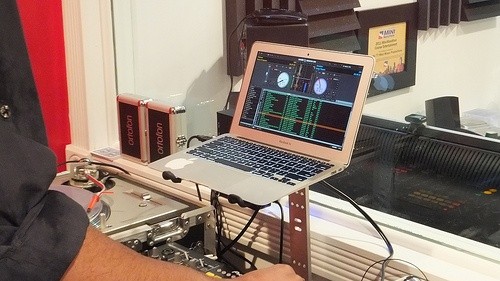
[0,0,305,281]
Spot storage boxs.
[114,92,151,164]
[144,100,187,162]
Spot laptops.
[148,41,375,207]
